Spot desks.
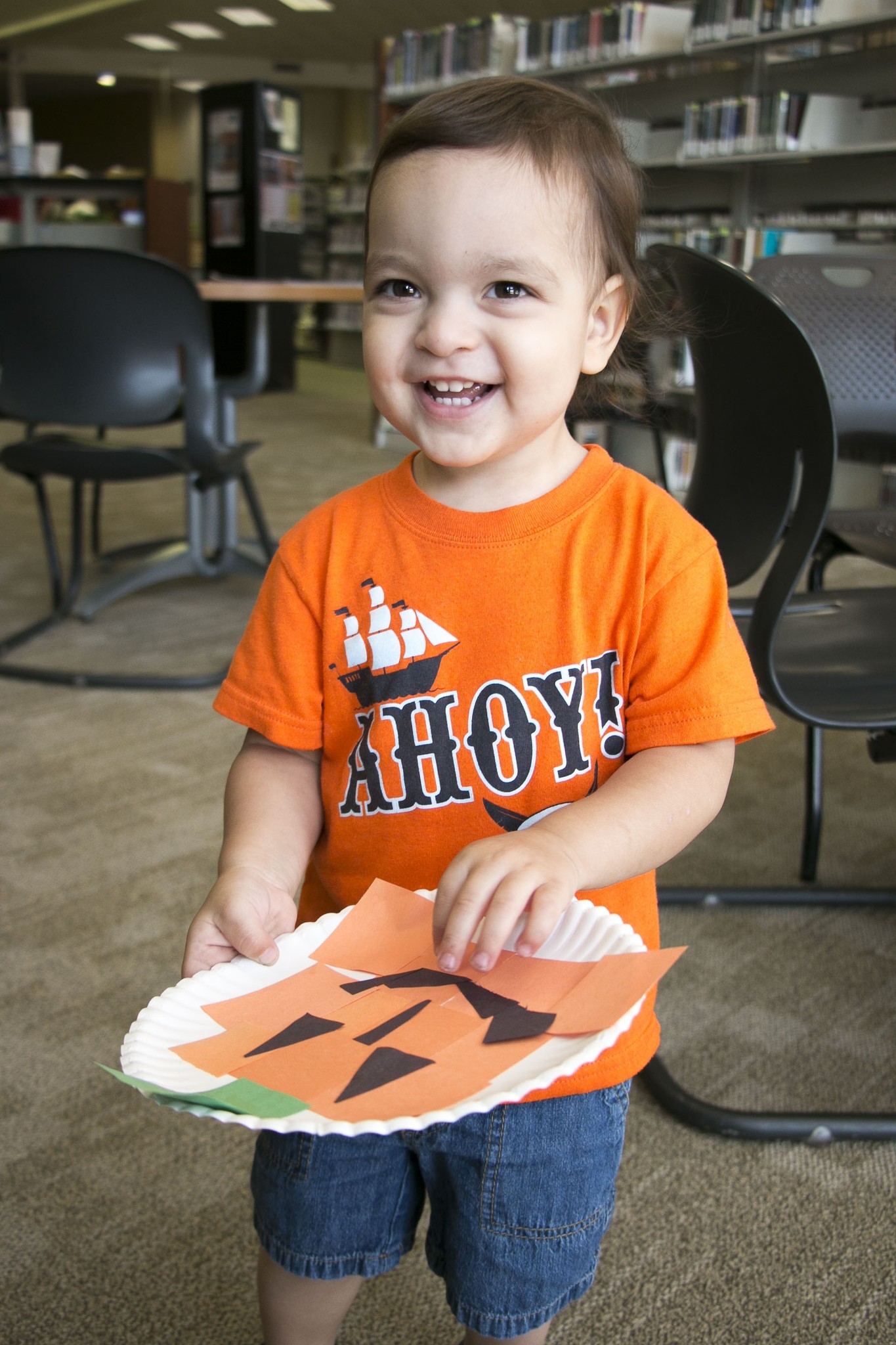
[87,266,367,559]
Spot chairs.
[742,247,896,888]
[1,238,290,699]
[628,240,896,1143]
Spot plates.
[115,883,650,1152]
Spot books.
[2,2,893,443]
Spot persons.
[174,72,782,1345]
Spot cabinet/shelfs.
[360,1,896,275]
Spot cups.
[6,106,63,177]
